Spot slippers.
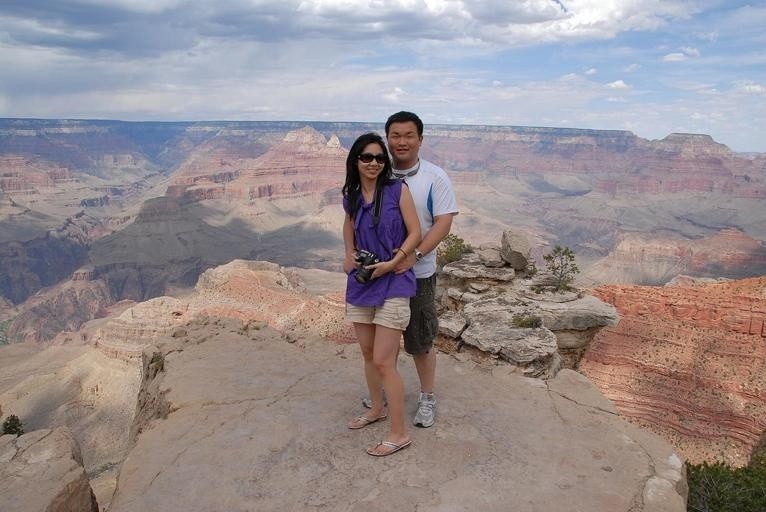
[346,414,386,430]
[366,440,412,456]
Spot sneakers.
[361,389,386,409]
[412,393,437,428]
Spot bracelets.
[397,247,408,259]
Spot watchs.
[413,246,423,261]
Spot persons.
[341,131,421,457]
[362,111,460,427]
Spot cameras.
[354,249,380,283]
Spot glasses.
[356,153,385,164]
[390,159,420,178]
